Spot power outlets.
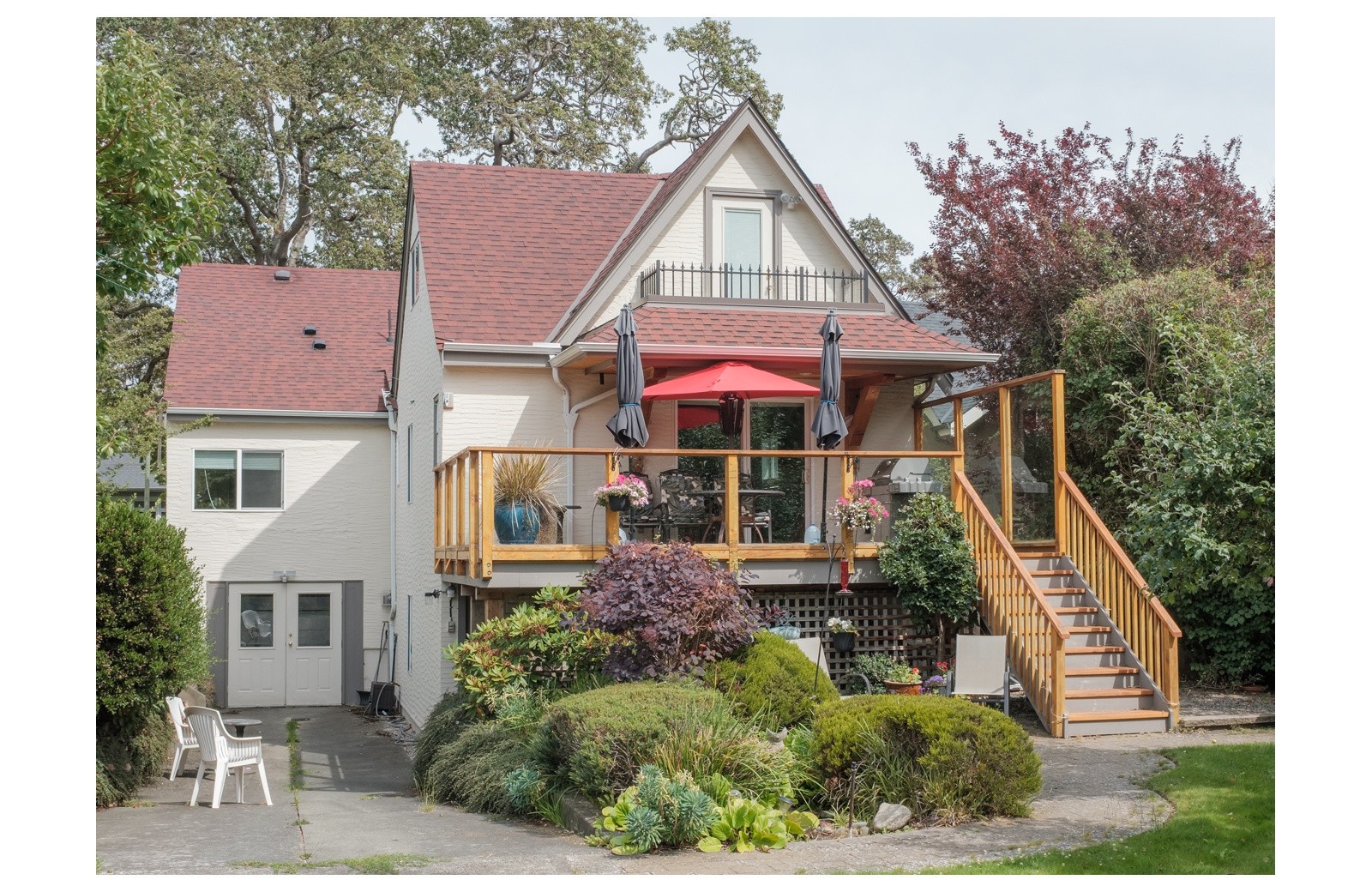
[447,620,456,633]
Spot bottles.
[804,524,821,543]
[619,527,626,542]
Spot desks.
[677,489,786,545]
[221,717,262,738]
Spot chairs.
[240,610,265,643]
[183,705,275,810]
[659,468,714,543]
[618,470,669,544]
[165,695,232,781]
[703,468,765,543]
[257,622,274,647]
[945,633,1010,717]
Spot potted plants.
[477,436,577,544]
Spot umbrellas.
[642,361,820,450]
[811,313,849,543]
[606,303,650,541]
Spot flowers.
[824,477,893,530]
[827,617,861,639]
[593,473,650,510]
[925,660,955,686]
[886,659,921,685]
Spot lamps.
[424,586,447,605]
[779,192,803,212]
[382,592,391,607]
[272,569,298,582]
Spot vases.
[847,507,867,528]
[926,683,947,697]
[607,493,631,513]
[830,631,857,653]
[883,677,923,697]
